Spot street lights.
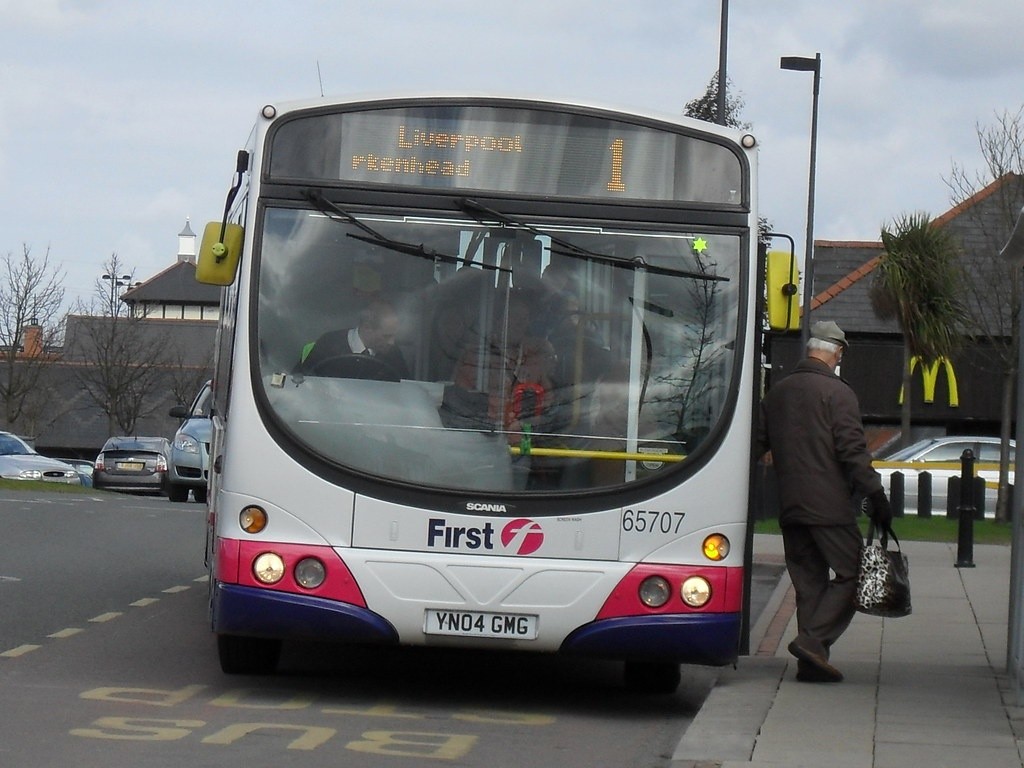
[101,273,132,318]
[777,53,825,372]
[116,281,142,318]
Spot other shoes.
[788,641,844,681]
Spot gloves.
[866,491,893,529]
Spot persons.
[426,251,607,490]
[299,298,410,382]
[755,319,892,684]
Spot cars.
[871,436,1016,516]
[52,457,95,489]
[169,379,219,503]
[91,435,172,496]
[0,431,81,487]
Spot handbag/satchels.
[856,517,912,617]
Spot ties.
[360,349,370,358]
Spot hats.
[810,321,849,355]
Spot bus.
[194,98,798,693]
